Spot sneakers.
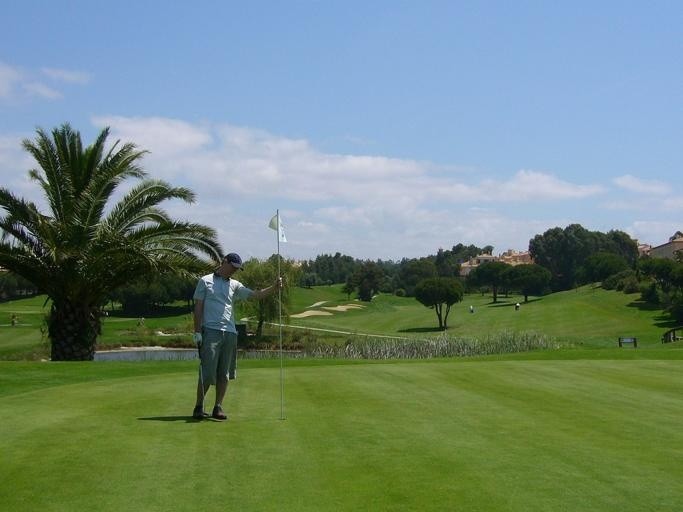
[193,407,211,419]
[212,406,227,419]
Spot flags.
[267,213,290,243]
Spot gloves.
[191,333,202,348]
[272,277,283,292]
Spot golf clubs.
[197,341,209,417]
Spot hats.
[224,252,244,271]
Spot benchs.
[618,337,637,347]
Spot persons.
[192,253,282,420]
